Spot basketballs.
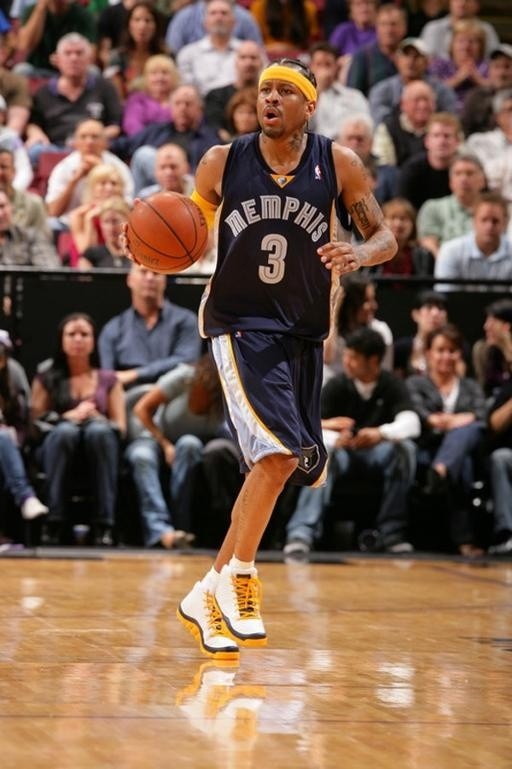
[126,191,207,274]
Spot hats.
[395,20,512,59]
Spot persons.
[0,0,512,558]
[117,55,399,662]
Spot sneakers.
[176,661,240,734]
[175,581,240,659]
[213,564,268,649]
[217,685,266,750]
